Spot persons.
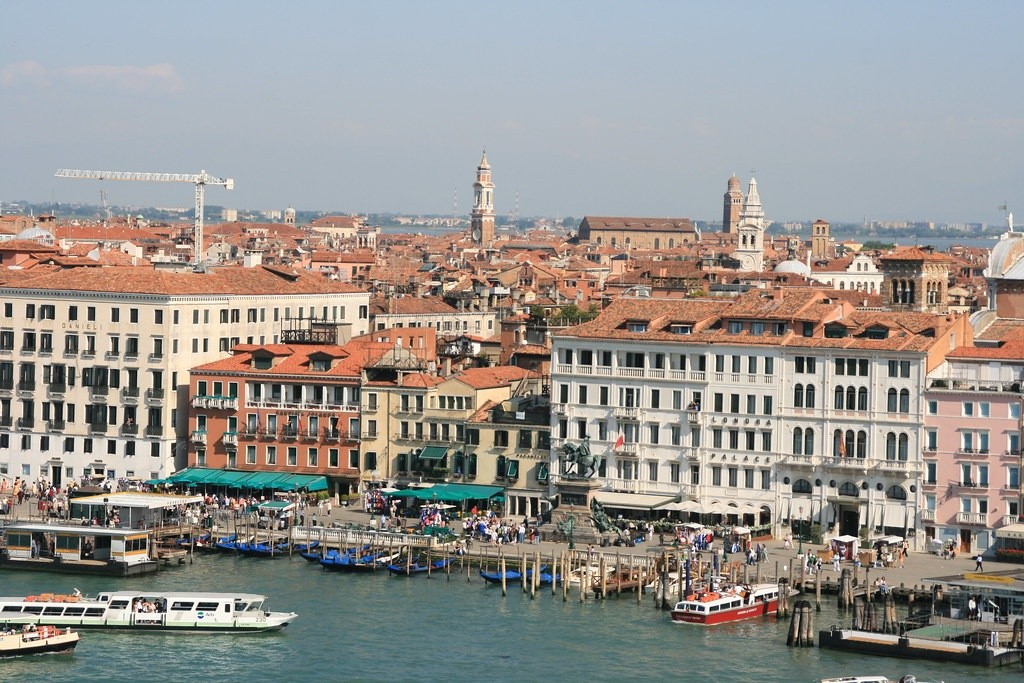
[366,503,983,621]
[570,434,591,464]
[0,476,331,559]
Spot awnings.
[380,483,504,501]
[419,445,450,460]
[145,467,331,492]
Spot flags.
[614,436,623,449]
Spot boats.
[479,558,615,588]
[0,623,85,658]
[298,543,456,576]
[178,528,320,555]
[643,568,800,627]
[0,524,160,578]
[0,592,300,635]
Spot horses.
[562,443,602,478]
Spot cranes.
[53,167,235,264]
